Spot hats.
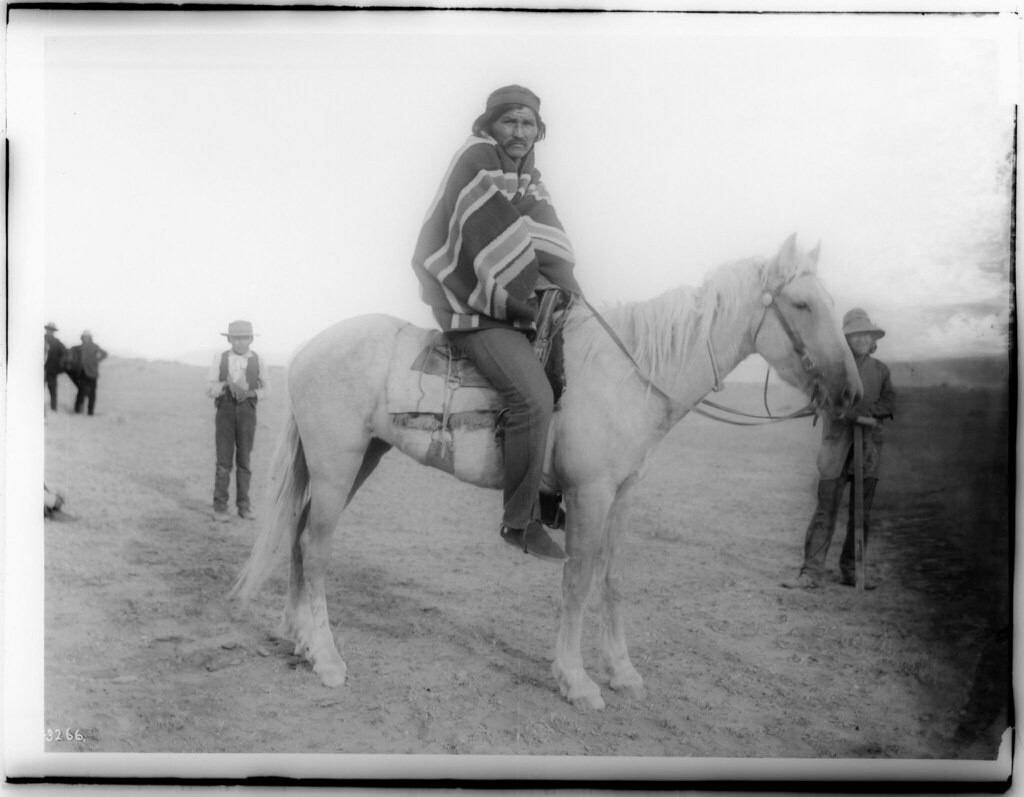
[221,321,261,338]
[45,322,56,330]
[843,308,885,339]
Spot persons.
[779,306,897,591]
[67,329,107,415]
[204,320,271,524]
[43,322,68,411]
[410,83,584,562]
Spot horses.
[228,232,865,711]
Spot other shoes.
[213,510,231,521]
[545,507,566,530]
[238,507,255,519]
[842,576,877,589]
[501,522,569,563]
[783,573,816,588]
